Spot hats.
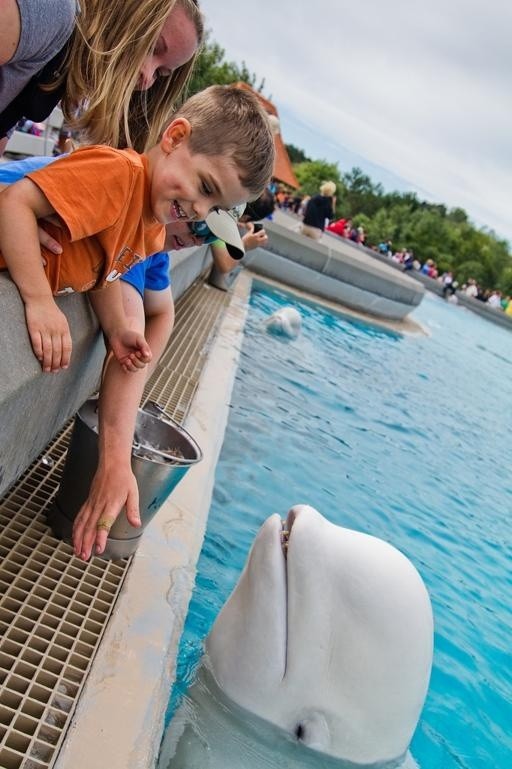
[204,203,247,260]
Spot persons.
[1,1,205,265]
[212,188,276,274]
[72,201,246,560]
[1,87,276,372]
[276,176,511,321]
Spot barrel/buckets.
[204,258,243,294]
[47,397,205,559]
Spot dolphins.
[155,501,436,769]
[263,307,303,340]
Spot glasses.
[188,219,218,244]
